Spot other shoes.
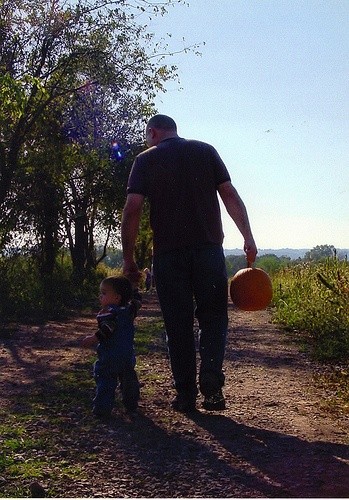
[173,395,197,411]
[204,391,225,410]
[93,407,112,417]
[122,398,138,411]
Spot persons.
[121,115,258,412]
[144,267,151,293]
[82,275,143,418]
